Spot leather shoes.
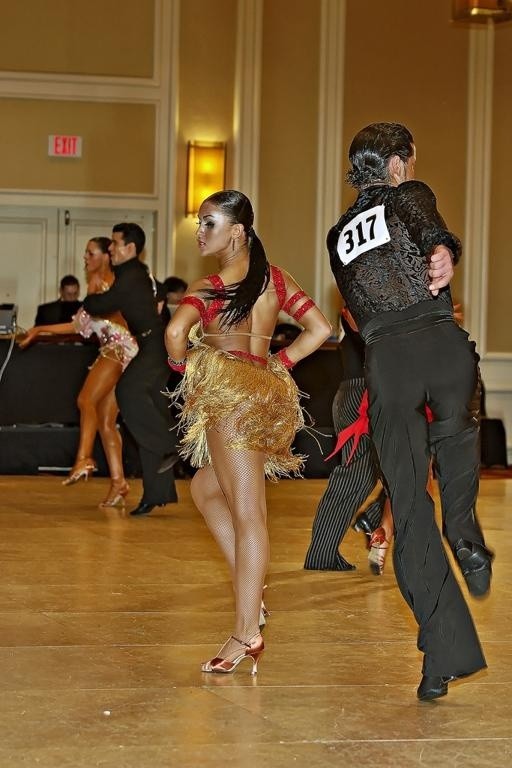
[452,538,496,597]
[129,499,167,515]
[416,664,488,701]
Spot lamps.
[185,138,228,218]
[452,0,512,25]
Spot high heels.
[365,527,392,576]
[352,511,374,542]
[60,456,95,487]
[199,600,269,674]
[98,476,130,511]
